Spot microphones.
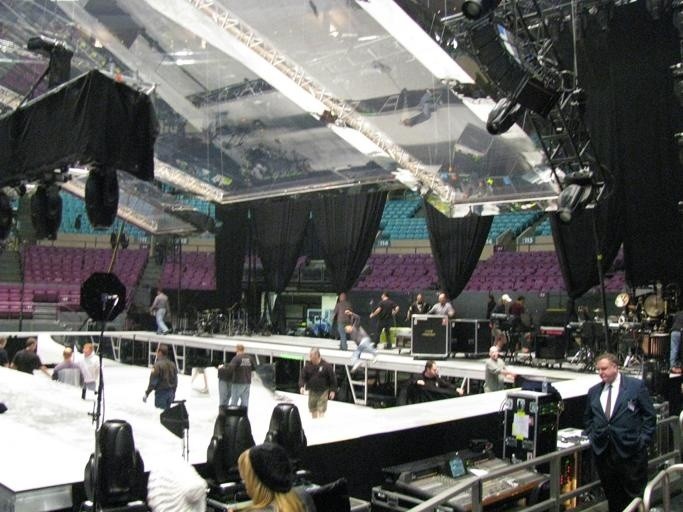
[101,293,118,302]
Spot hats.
[502,294,512,302]
[147,456,207,512]
[250,443,293,492]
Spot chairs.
[263,403,317,483]
[81,417,146,512]
[201,403,255,494]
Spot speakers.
[537,335,567,359]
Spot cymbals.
[644,295,665,318]
[615,292,629,307]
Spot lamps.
[557,169,599,223]
[31,170,62,241]
[486,96,525,135]
[0,184,13,241]
[459,1,502,23]
[83,163,121,229]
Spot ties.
[604,384,612,421]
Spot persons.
[1,292,530,512]
[151,288,173,336]
[403,90,444,128]
[250,160,270,184]
[665,311,683,375]
[579,353,658,511]
[442,163,488,198]
